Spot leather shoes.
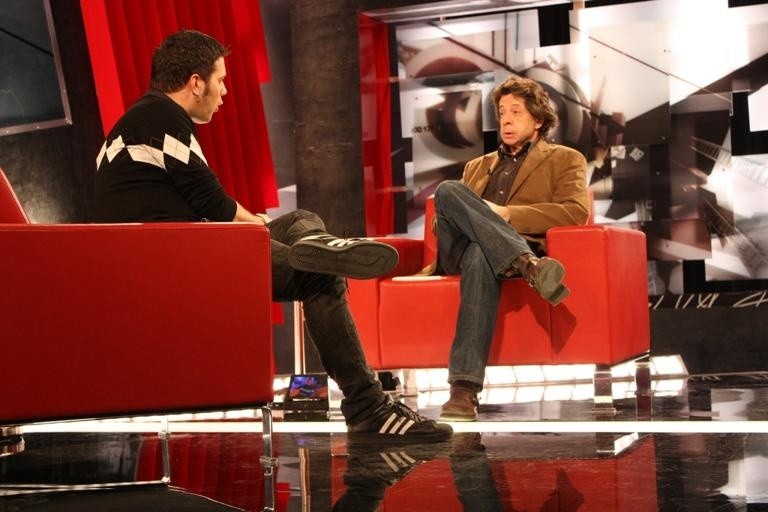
[440,386,480,421]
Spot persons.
[89,27,452,440]
[433,72,594,422]
[328,440,450,511]
[446,430,502,512]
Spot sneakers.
[287,230,399,279]
[347,394,454,443]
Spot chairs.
[347,195,651,404]
[0,168,273,444]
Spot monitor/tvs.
[284,373,329,411]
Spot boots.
[517,251,570,307]
